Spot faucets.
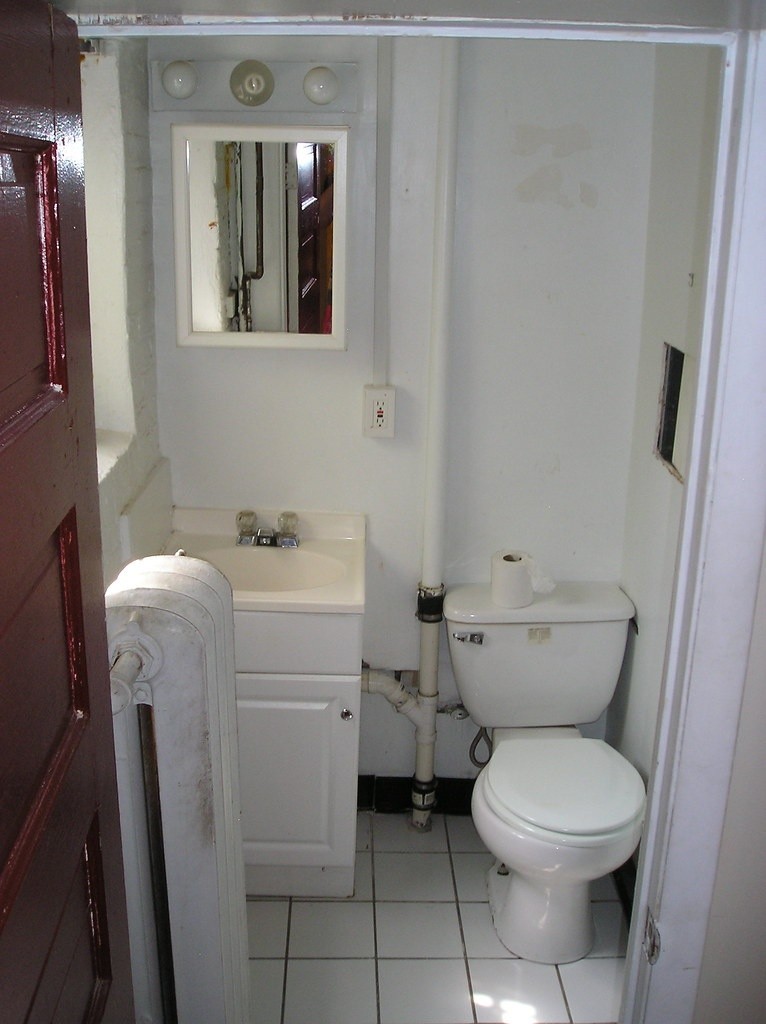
[256,528,276,546]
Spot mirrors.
[170,122,350,349]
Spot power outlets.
[362,385,396,438]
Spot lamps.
[151,59,360,114]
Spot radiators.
[104,549,253,1024]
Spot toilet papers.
[490,547,556,609]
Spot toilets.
[442,579,647,966]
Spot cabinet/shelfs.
[233,611,363,898]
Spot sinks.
[176,538,350,601]
